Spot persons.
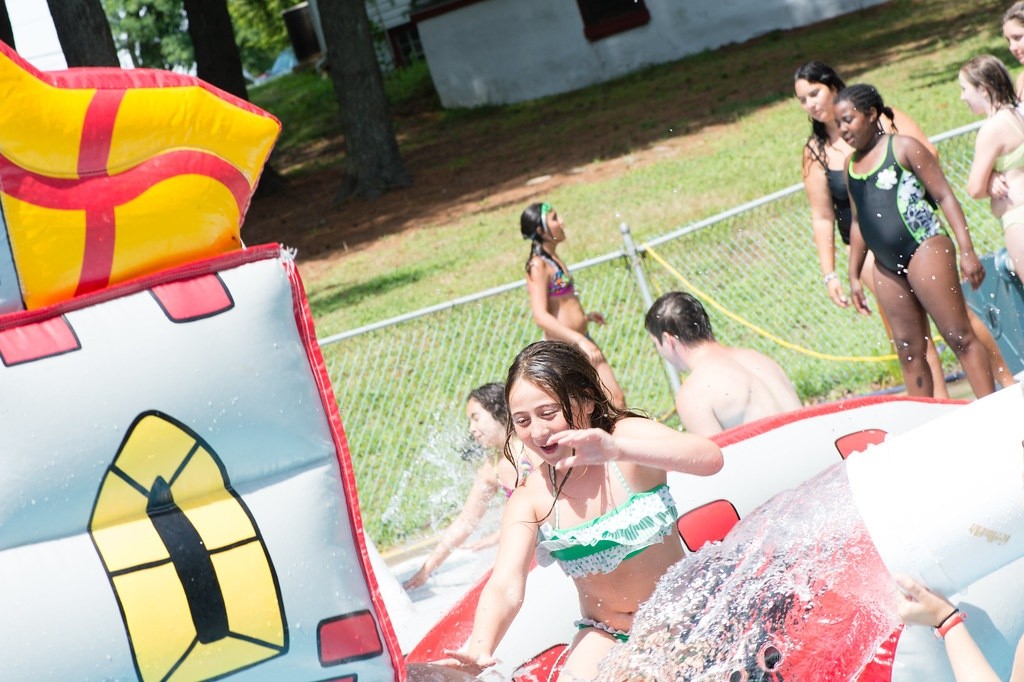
[403,382,546,591]
[959,54,1024,285]
[1001,0,1024,103]
[832,83,995,400]
[644,291,806,438]
[794,60,947,398]
[892,574,1024,682]
[520,202,630,412]
[435,340,725,682]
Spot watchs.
[931,612,966,642]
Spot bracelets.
[935,609,959,629]
[824,272,837,283]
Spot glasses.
[541,203,553,235]
